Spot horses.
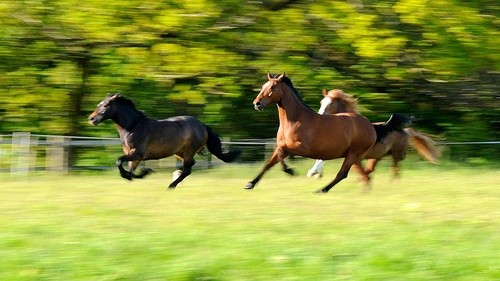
[244,71,441,194]
[88,94,242,189]
